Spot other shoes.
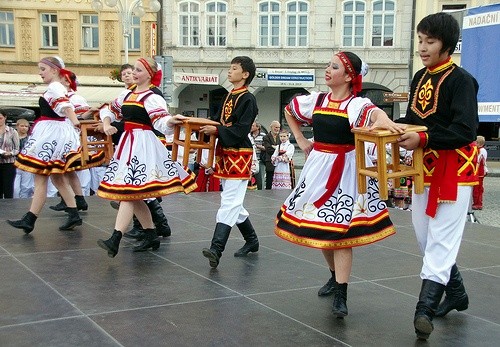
[471,204,482,210]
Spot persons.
[13,119,413,211]
[0,111,21,199]
[108,62,163,210]
[49,69,88,212]
[93,83,172,241]
[471,135,488,210]
[390,12,481,342]
[200,55,260,269]
[274,51,405,319]
[6,55,85,234]
[98,54,191,259]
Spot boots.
[123,213,142,238]
[97,228,123,258]
[234,217,259,258]
[202,222,232,268]
[49,196,69,212]
[74,195,88,210]
[147,199,171,237]
[6,210,38,234]
[318,268,337,296]
[130,228,160,251]
[110,200,120,209]
[59,207,82,230]
[331,282,348,320]
[413,279,446,340]
[433,263,469,319]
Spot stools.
[171,118,221,167]
[92,103,109,119]
[351,123,428,200]
[79,120,113,166]
